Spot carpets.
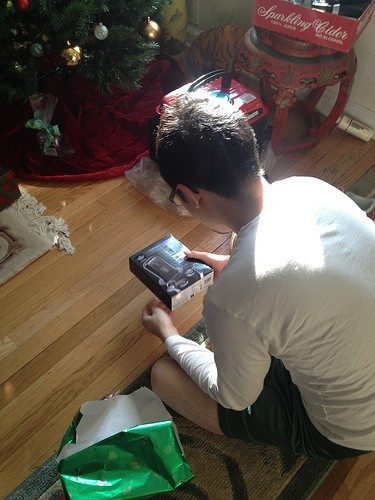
[0,184,75,285]
[4,164,375,499]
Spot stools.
[230,25,358,154]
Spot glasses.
[168,186,199,206]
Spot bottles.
[336,115,375,142]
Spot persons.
[141,91,375,462]
